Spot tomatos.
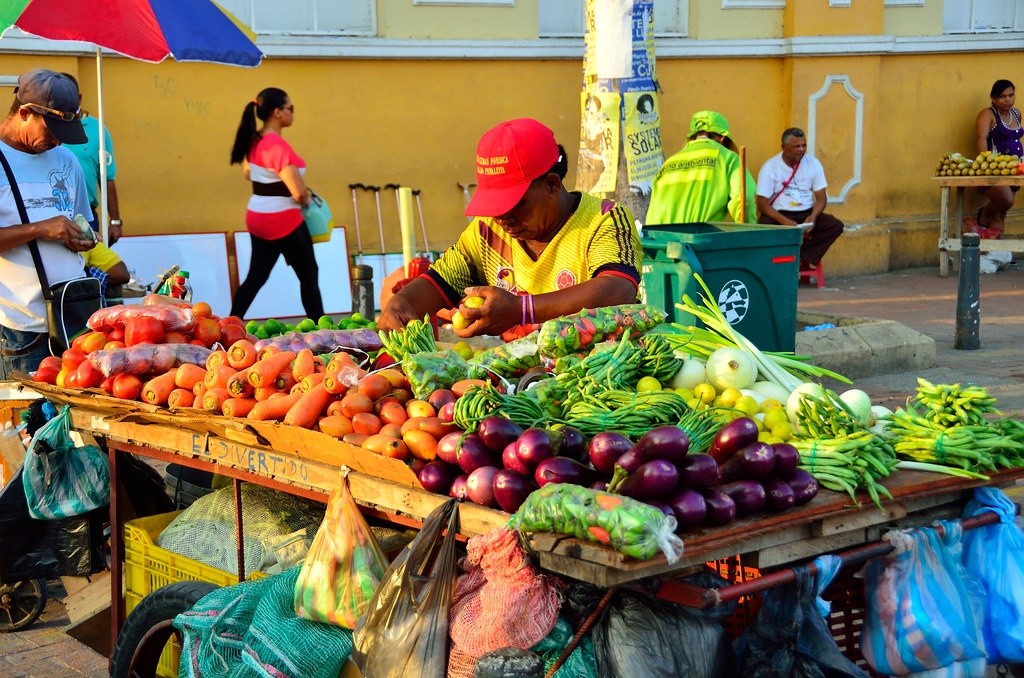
[34,294,258,400]
[318,369,486,463]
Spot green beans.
[882,377,1024,474]
[455,327,701,441]
[781,384,904,512]
[377,314,437,360]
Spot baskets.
[703,552,891,678]
[124,512,402,678]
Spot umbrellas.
[0,0,265,251]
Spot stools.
[799,258,823,288]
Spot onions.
[663,345,898,439]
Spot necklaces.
[999,110,1013,126]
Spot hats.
[687,111,739,153]
[465,118,559,217]
[13,69,88,144]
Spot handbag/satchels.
[301,188,334,243]
[351,495,465,678]
[757,213,768,224]
[22,405,111,519]
[45,276,103,358]
[293,465,390,631]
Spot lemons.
[246,313,377,339]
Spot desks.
[933,176,1024,276]
[58,405,1024,677]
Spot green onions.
[894,459,990,481]
[645,271,852,394]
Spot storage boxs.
[706,560,886,677]
[123,509,271,676]
[0,382,47,492]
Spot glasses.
[19,103,82,121]
[282,105,293,113]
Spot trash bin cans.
[639,222,802,356]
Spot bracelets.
[110,219,122,227]
[528,294,534,324]
[521,296,526,327]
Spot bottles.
[158,269,193,303]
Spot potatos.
[934,152,1020,176]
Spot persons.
[378,118,644,346]
[756,128,844,287]
[645,110,758,226]
[0,68,98,382]
[229,88,326,325]
[70,234,130,345]
[967,79,1024,239]
[57,72,123,248]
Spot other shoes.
[798,261,811,285]
[977,207,988,229]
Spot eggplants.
[420,417,818,527]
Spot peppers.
[510,483,662,559]
[402,305,668,396]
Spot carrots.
[142,340,368,432]
